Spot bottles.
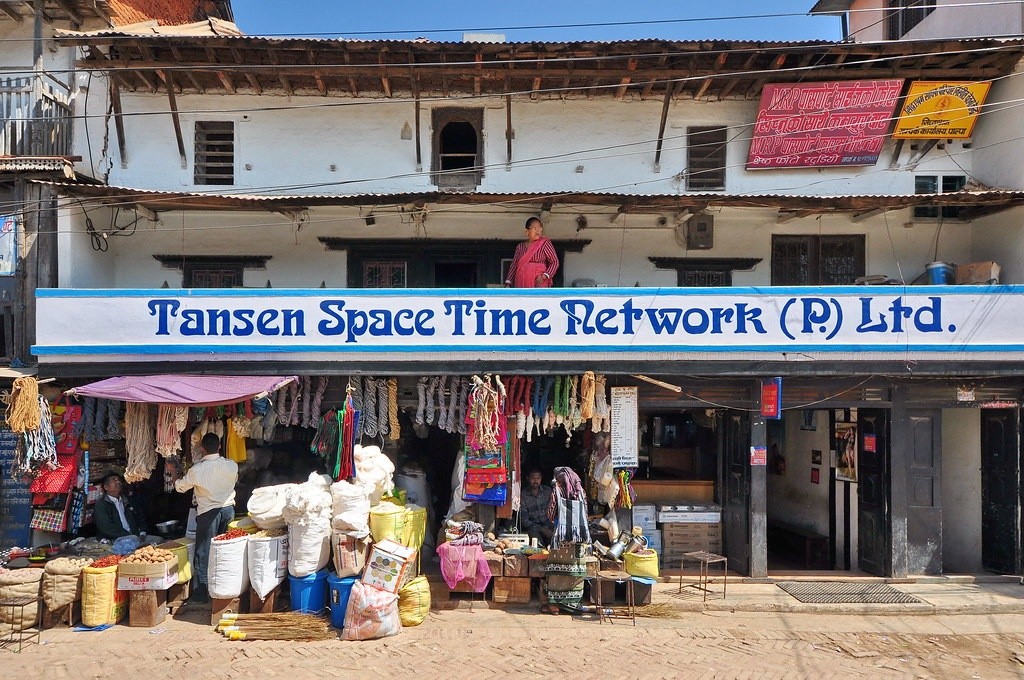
[606,526,648,561]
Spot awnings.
[61,373,297,405]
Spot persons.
[91,474,164,545]
[844,426,855,479]
[505,217,559,288]
[519,467,556,549]
[540,467,592,615]
[165,432,238,603]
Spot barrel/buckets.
[327,571,362,628]
[287,567,330,614]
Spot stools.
[679,551,727,602]
[596,571,636,627]
[0,595,43,654]
